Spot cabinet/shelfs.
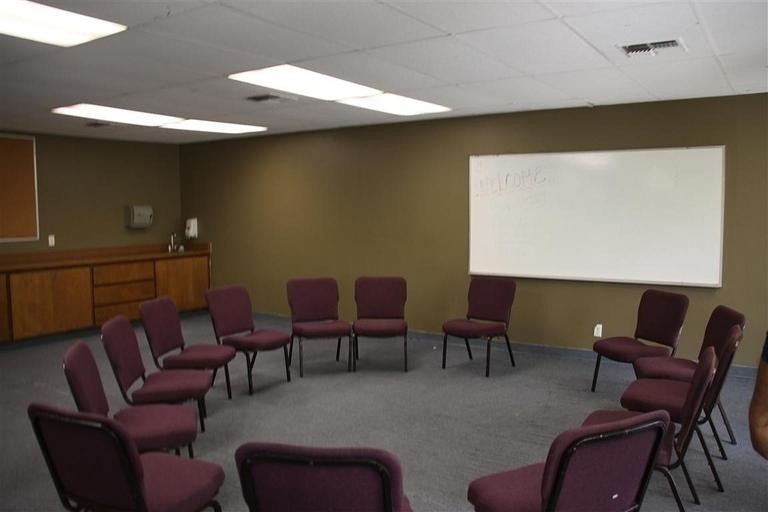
[0,273,10,342]
[155,256,209,313]
[10,266,93,341]
[92,261,156,327]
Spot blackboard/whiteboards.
[469,145,726,287]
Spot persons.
[748,328,768,460]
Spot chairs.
[467,410,671,512]
[100,314,213,456]
[633,305,745,444]
[205,286,292,395]
[581,346,719,512]
[286,277,352,377]
[352,276,407,372]
[442,278,516,377]
[621,324,743,492]
[28,403,225,512]
[591,289,689,392]
[138,298,236,418]
[234,442,413,512]
[62,340,198,459]
